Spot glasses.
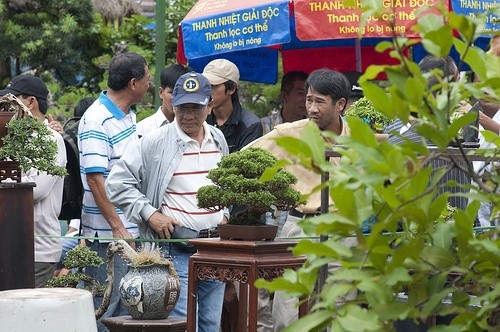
[173,103,208,117]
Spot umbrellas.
[177,0,500,85]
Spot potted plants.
[0,93,69,179]
[44,240,181,319]
[344,93,397,142]
[196,147,307,242]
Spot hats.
[0,74,49,100]
[344,71,365,96]
[172,71,211,107]
[200,59,239,86]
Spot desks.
[102,315,187,332]
[0,182,37,290]
[187,238,309,332]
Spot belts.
[290,208,322,219]
[199,227,218,238]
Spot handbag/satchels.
[57,117,83,220]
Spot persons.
[77,53,151,332]
[47,97,98,289]
[239,69,367,332]
[259,71,309,237]
[343,54,500,296]
[202,59,272,332]
[105,71,230,332]
[0,74,67,288]
[136,64,195,139]
